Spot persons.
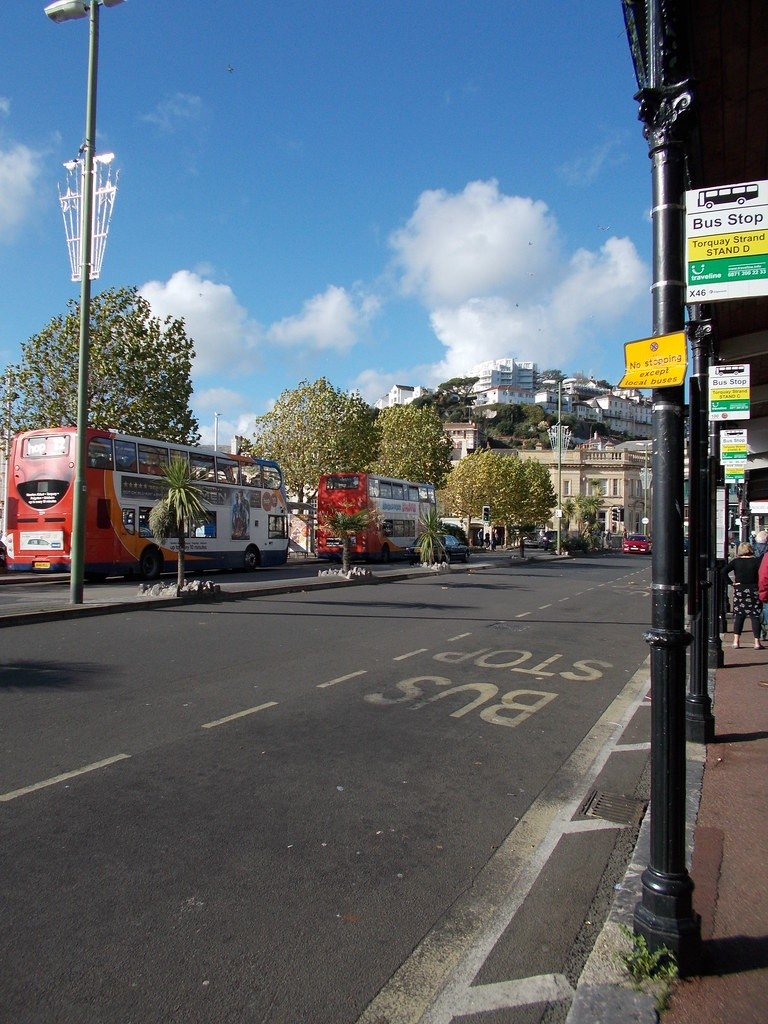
[485,530,490,548]
[478,529,484,547]
[243,475,254,486]
[606,530,612,552]
[492,528,498,550]
[722,542,766,649]
[233,492,250,539]
[624,528,627,542]
[749,531,768,561]
[758,552,768,688]
[256,474,268,487]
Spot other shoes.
[731,644,739,649]
[754,644,765,649]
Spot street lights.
[214,411,223,451]
[43,0,121,604]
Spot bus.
[316,472,437,564]
[5,428,292,582]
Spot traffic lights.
[611,506,619,522]
[482,506,491,523]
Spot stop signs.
[642,518,649,524]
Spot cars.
[623,534,649,554]
[403,531,470,565]
